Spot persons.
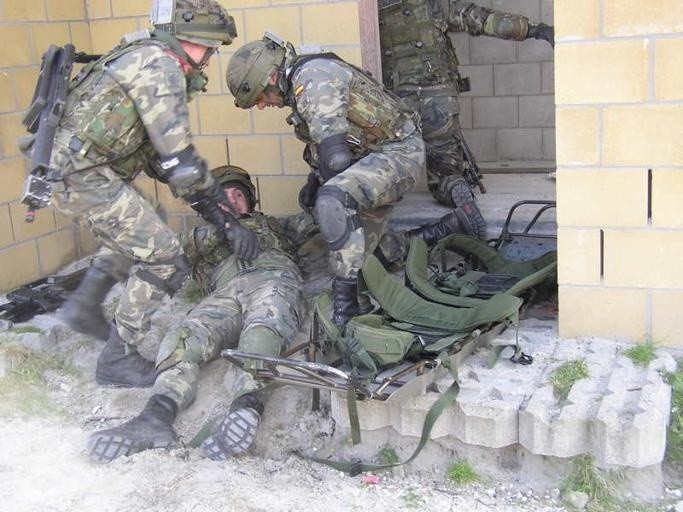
[378,0,554,243]
[83,165,321,466]
[225,31,425,369]
[18,0,257,388]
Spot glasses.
[190,16,236,38]
[212,165,249,179]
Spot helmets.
[151,0,237,45]
[209,164,256,194]
[225,31,286,110]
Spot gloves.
[225,216,260,262]
[533,23,553,46]
[297,172,319,212]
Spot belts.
[66,135,97,161]
[397,83,448,91]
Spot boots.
[95,321,157,389]
[87,394,179,463]
[58,264,117,340]
[331,277,359,330]
[199,394,266,460]
[406,207,473,250]
[448,182,487,241]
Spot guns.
[0,267,89,323]
[22,43,75,222]
[459,137,487,195]
